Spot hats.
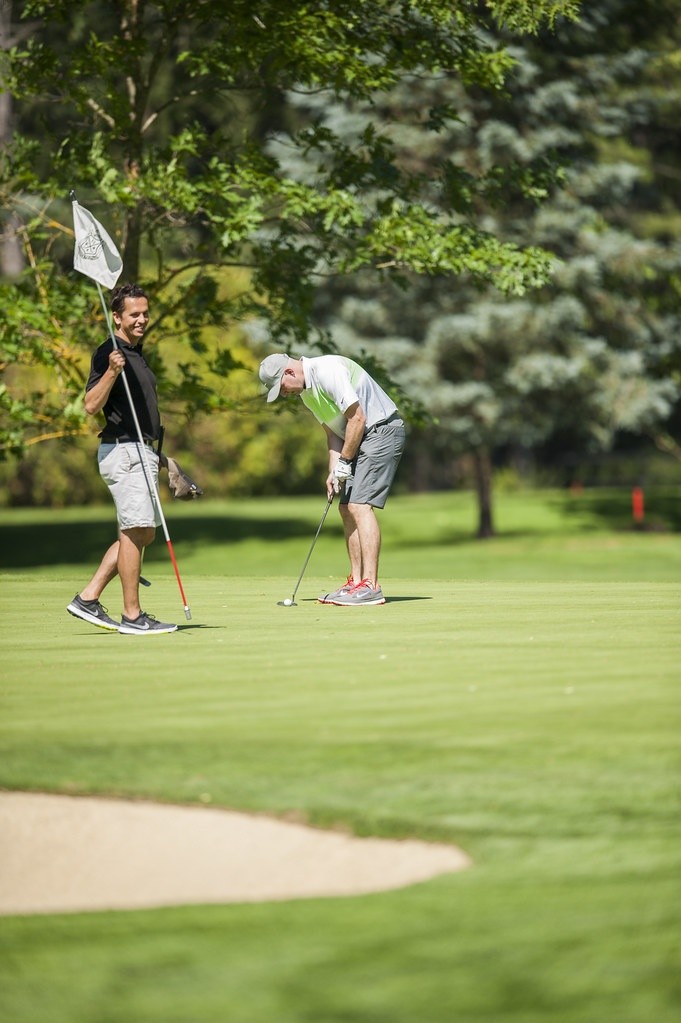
[166,457,203,501]
[259,353,289,402]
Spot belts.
[100,437,152,447]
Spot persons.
[258,350,407,608]
[66,283,183,635]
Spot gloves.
[332,458,353,491]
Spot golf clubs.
[277,485,340,606]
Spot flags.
[72,201,124,290]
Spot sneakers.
[68,595,178,634]
[319,581,385,606]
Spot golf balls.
[284,599,292,606]
